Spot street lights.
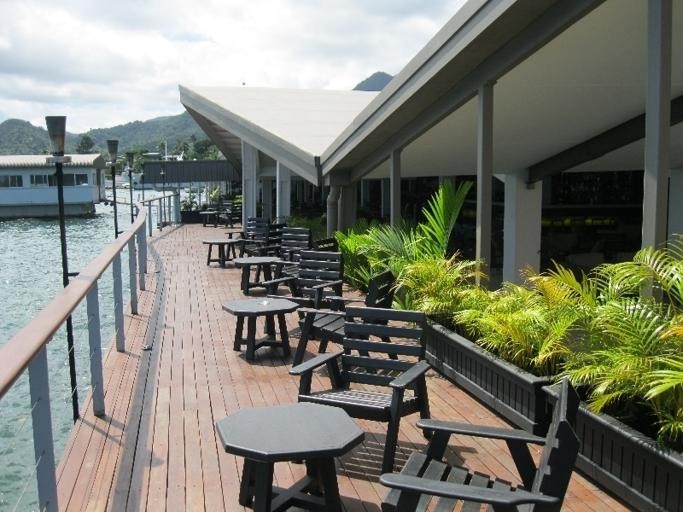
[105,138,120,239]
[43,115,82,424]
[125,151,136,224]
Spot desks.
[222,297,298,360]
[216,402,365,512]
[203,238,244,268]
